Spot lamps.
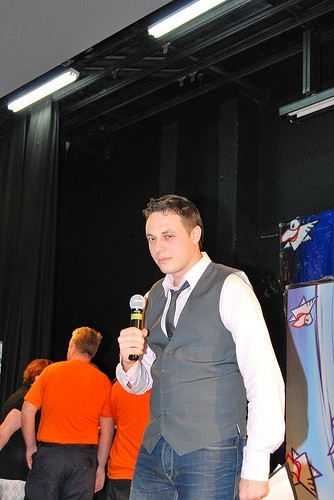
[279,22,334,121]
[147,0,226,38]
[7,68,79,113]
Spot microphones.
[129,295,146,360]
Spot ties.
[166,280,190,340]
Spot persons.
[116,195,286,500]
[21,327,113,500]
[0,359,53,500]
[107,379,152,500]
